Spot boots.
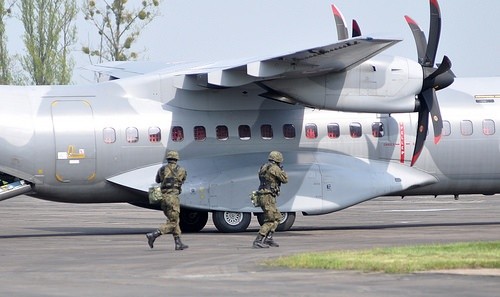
[146,229,162,248]
[263,231,279,247]
[253,233,269,248]
[174,236,188,250]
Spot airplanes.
[1,2,500,232]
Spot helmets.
[268,151,283,162]
[166,151,179,160]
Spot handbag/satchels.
[149,186,162,204]
[251,191,260,207]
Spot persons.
[250,148,290,247]
[145,150,190,253]
